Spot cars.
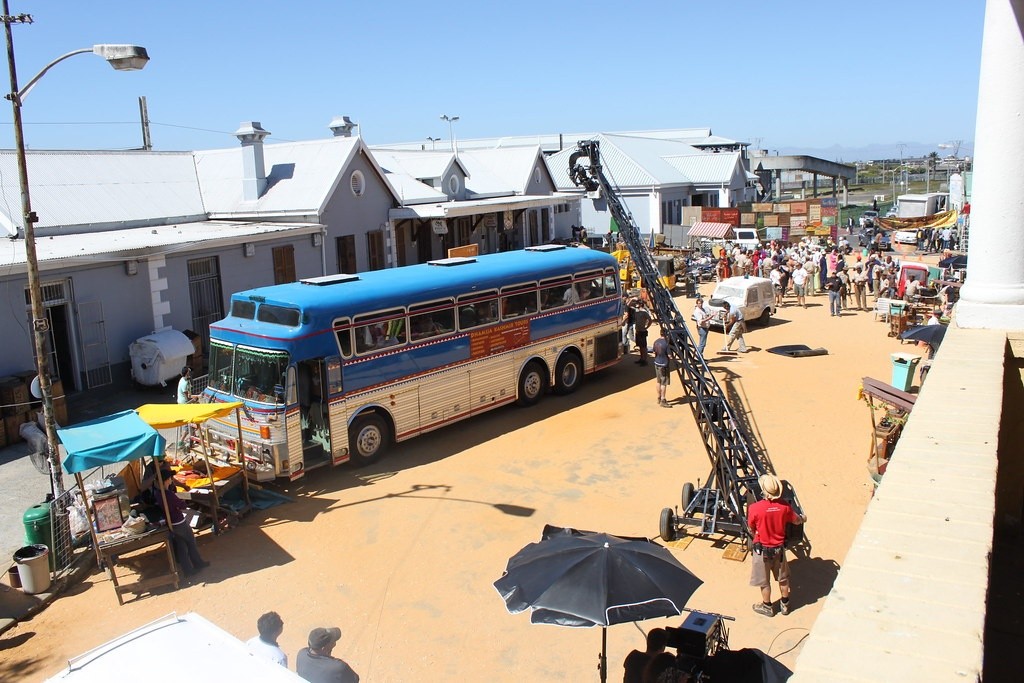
[893,228,918,244]
[857,227,892,250]
[859,210,880,226]
[691,276,776,328]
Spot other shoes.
[775,302,782,307]
[780,599,792,615]
[657,397,672,408]
[803,305,807,309]
[862,308,868,312]
[193,442,198,444]
[795,303,801,306]
[855,308,861,311]
[634,358,649,366]
[177,440,183,449]
[194,559,209,568]
[184,567,200,578]
[752,602,775,617]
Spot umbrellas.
[493,524,704,683]
[647,228,656,249]
[901,324,947,345]
[938,255,967,269]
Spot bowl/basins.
[126,518,146,533]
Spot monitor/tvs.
[665,627,706,658]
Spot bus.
[195,244,622,483]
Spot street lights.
[9,44,150,561]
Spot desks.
[92,517,180,606]
[163,449,252,535]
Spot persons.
[848,215,958,253]
[177,366,203,450]
[716,236,956,316]
[748,473,807,618]
[619,292,652,367]
[721,300,748,353]
[653,328,673,408]
[570,224,614,249]
[296,627,359,683]
[694,298,718,364]
[622,628,666,683]
[959,200,970,228]
[143,456,210,577]
[928,309,943,359]
[243,610,288,668]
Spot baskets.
[123,517,146,534]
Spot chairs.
[873,297,892,325]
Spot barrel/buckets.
[8,566,22,587]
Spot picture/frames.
[91,493,123,532]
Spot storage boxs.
[186,354,203,373]
[0,370,67,446]
[182,329,202,357]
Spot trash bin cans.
[13,538,53,595]
[891,353,922,395]
[23,500,58,574]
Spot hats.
[156,469,176,481]
[843,267,848,271]
[758,473,783,500]
[307,627,341,650]
[856,256,861,261]
[632,301,643,304]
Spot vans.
[702,227,760,250]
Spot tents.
[134,401,248,505]
[56,410,173,565]
[687,222,736,247]
